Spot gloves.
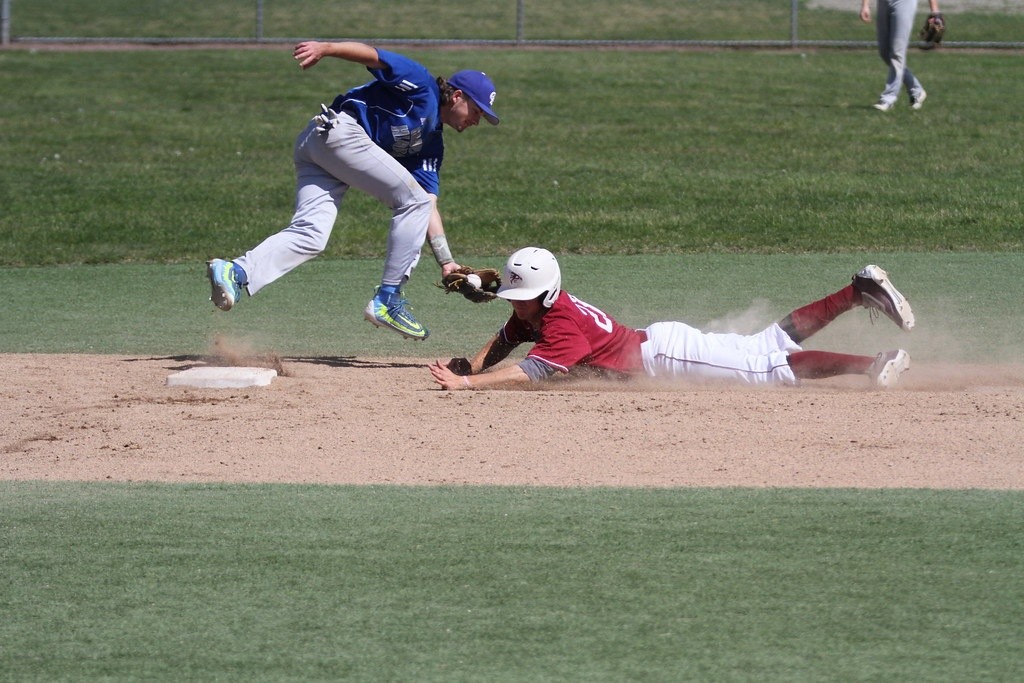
[312,103,342,136]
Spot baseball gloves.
[441,357,473,393]
[915,14,946,53]
[442,265,501,303]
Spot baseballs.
[466,274,482,288]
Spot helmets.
[496,245,562,308]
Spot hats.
[448,70,500,127]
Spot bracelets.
[463,376,469,384]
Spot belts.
[638,329,646,345]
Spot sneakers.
[912,89,925,108]
[362,284,429,340]
[852,264,914,331]
[868,348,910,389]
[203,257,241,310]
[872,100,895,111]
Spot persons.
[428,247,914,390]
[206,41,501,339]
[860,0,945,113]
